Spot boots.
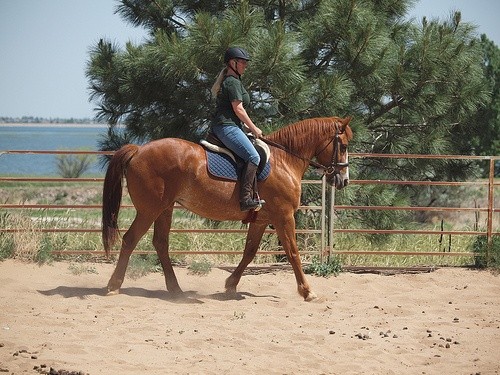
[239,161,266,211]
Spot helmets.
[224,47,252,64]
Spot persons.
[210,46,266,212]
